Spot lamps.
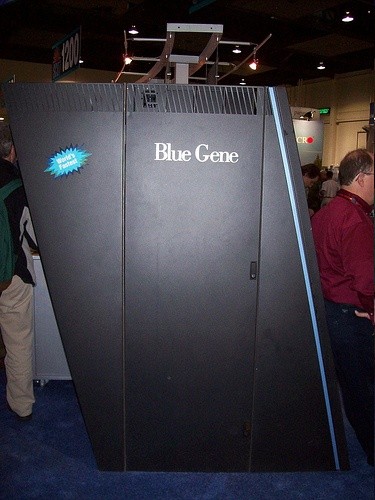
[249,49,259,71]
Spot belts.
[325,305,358,314]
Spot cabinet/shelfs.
[32,254,73,386]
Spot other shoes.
[3,399,32,420]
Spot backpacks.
[0,178,23,293]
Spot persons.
[299,163,341,217]
[0,125,38,423]
[309,148,375,469]
